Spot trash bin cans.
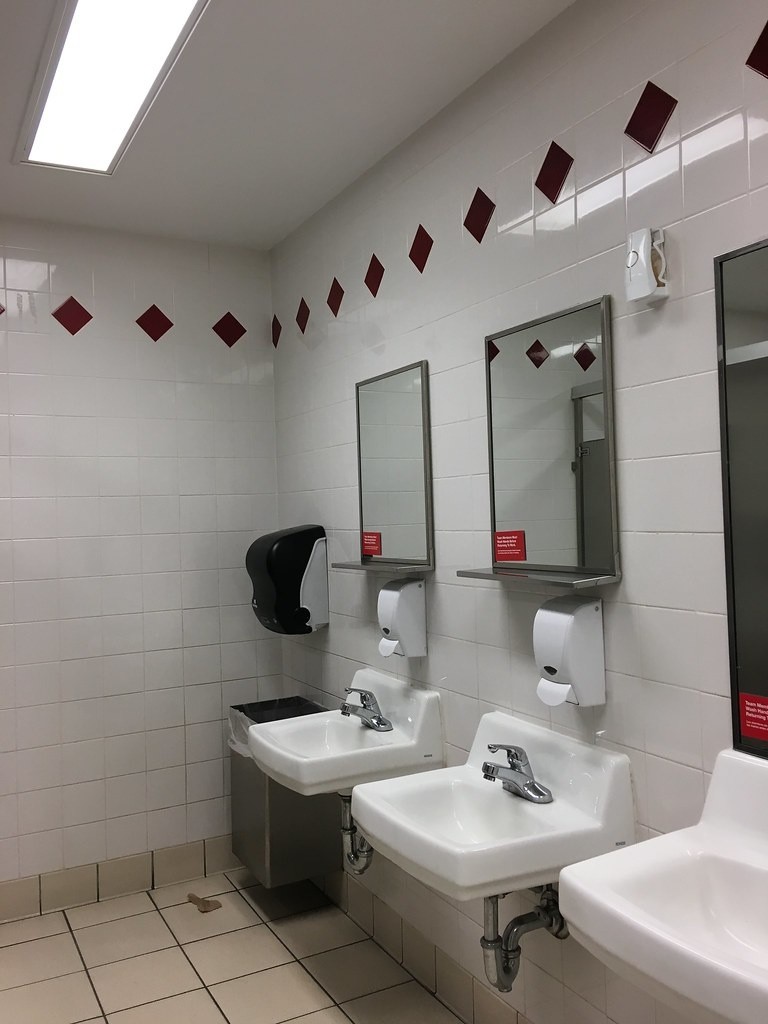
[228,694,344,890]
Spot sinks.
[558,823,767,1023]
[247,709,414,796]
[349,764,595,902]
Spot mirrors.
[712,238,768,760]
[329,359,435,565]
[456,294,621,575]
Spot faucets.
[481,743,554,804]
[338,686,394,732]
